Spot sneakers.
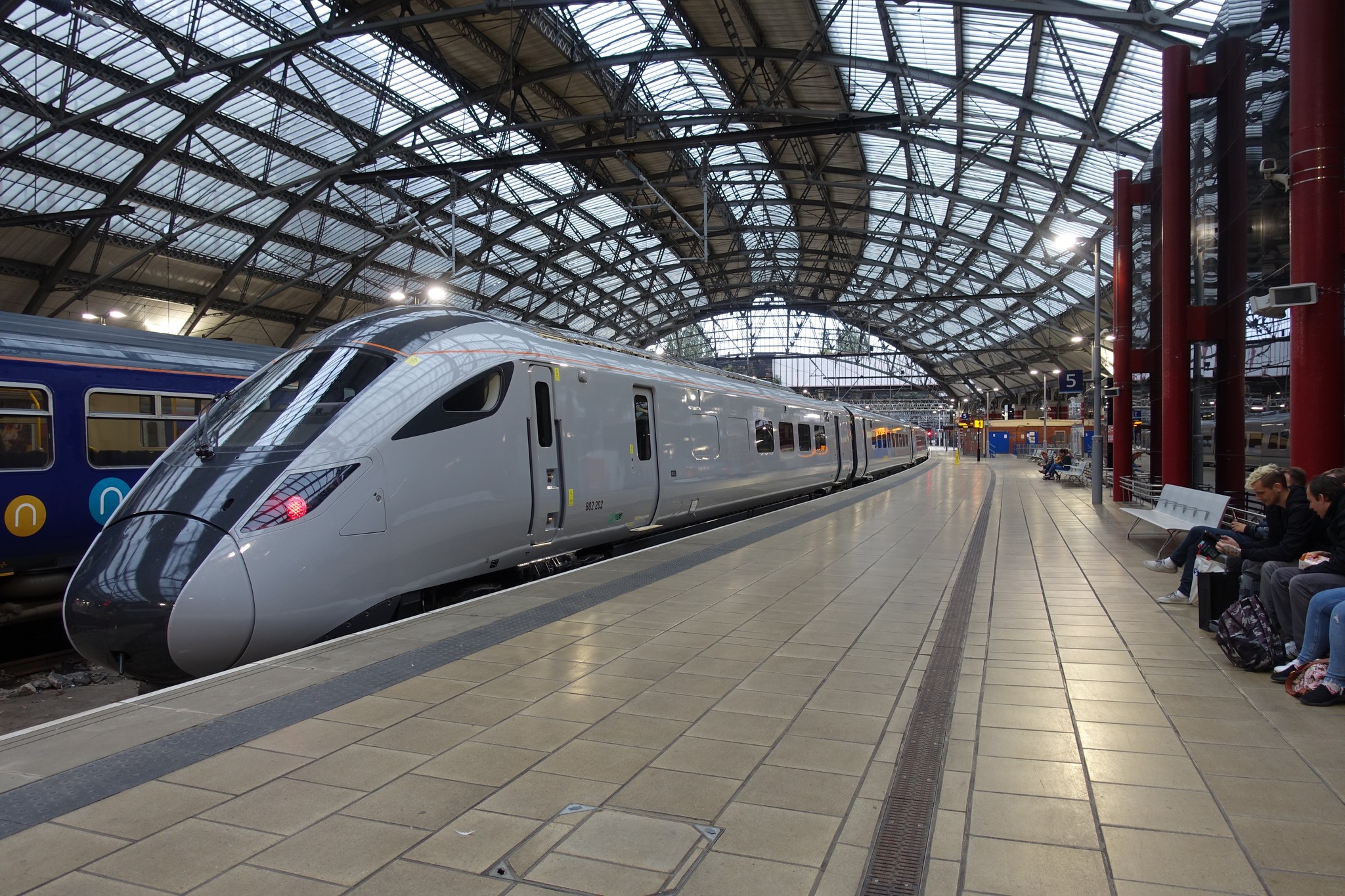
[1156,592,1191,604]
[1144,558,1178,573]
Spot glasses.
[1041,451,1046,455]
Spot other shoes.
[1047,478,1054,481]
[1273,659,1300,673]
[1209,620,1219,632]
[1299,683,1345,707]
[1270,664,1299,682]
[1041,477,1050,480]
[1043,475,1050,477]
[1038,470,1046,474]
[1285,640,1299,659]
[1056,475,1060,480]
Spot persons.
[1215,464,1322,631]
[1143,468,1307,603]
[1271,475,1345,658]
[1271,587,1344,709]
[1037,448,1073,481]
[1259,468,1345,632]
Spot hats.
[1134,451,1142,456]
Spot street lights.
[1071,335,1116,389]
[976,388,999,459]
[950,399,968,459]
[933,405,954,452]
[1030,369,1061,455]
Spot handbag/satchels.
[1196,570,1240,633]
[1286,657,1329,696]
[1188,550,1226,603]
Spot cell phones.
[1204,530,1222,541]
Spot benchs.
[1026,449,1054,464]
[1119,484,1232,559]
[1054,457,1075,482]
[1061,461,1087,487]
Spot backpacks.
[1215,595,1287,671]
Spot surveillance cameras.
[1259,158,1277,174]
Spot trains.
[61,272,928,679]
[0,309,363,600]
